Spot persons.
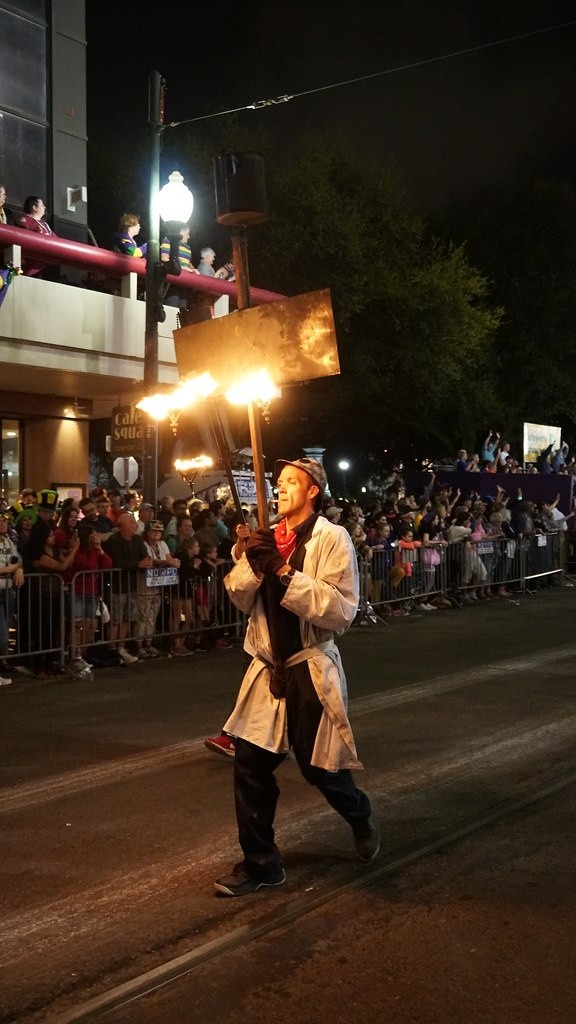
[0,428,576,693]
[106,214,145,293]
[0,185,12,287]
[19,197,59,275]
[201,459,383,896]
[161,224,200,309]
[213,258,237,285]
[197,243,217,314]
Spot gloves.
[245,527,286,575]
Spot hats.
[145,519,164,532]
[274,456,327,511]
[32,490,63,511]
[139,503,153,509]
[96,598,110,623]
[14,510,36,528]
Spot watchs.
[280,568,296,585]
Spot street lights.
[339,460,349,499]
[142,171,193,518]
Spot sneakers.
[352,814,380,861]
[214,862,285,895]
[204,734,237,758]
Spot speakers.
[212,153,268,217]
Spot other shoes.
[1,634,246,686]
[353,592,515,624]
